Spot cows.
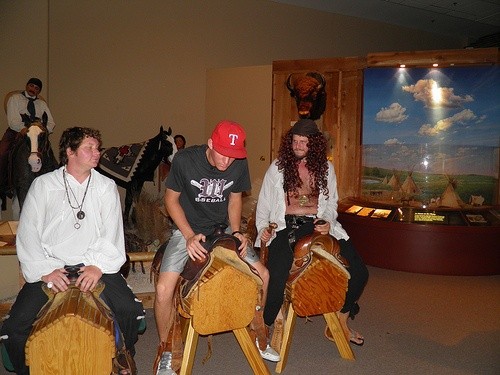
[284,70,326,120]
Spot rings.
[47,282,53,288]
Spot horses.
[56,124,180,230]
[0,110,60,220]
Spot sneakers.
[255,337,280,362]
[156,352,178,375]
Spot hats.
[291,119,318,136]
[27,78,42,89]
[209,120,248,158]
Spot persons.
[255,118,368,345]
[0,79,56,209]
[0,127,146,375]
[154,119,281,375]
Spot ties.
[21,91,37,121]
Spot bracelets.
[232,231,243,235]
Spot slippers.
[350,330,364,346]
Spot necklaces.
[61,166,93,229]
